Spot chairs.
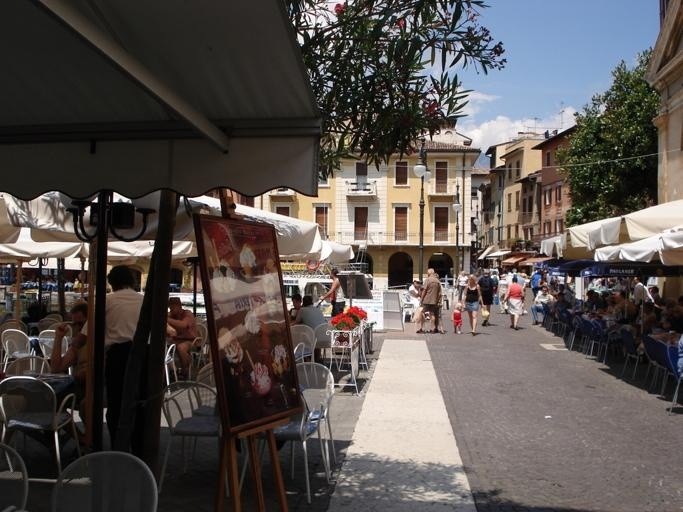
[541,302,683,415]
[0,283,338,512]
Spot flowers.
[347,307,368,324]
[330,313,357,333]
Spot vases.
[335,333,349,346]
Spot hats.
[482,308,489,321]
[168,297,180,303]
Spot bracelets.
[327,292,329,296]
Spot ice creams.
[239,243,258,278]
[271,346,287,376]
[244,309,261,335]
[222,333,242,376]
[244,346,274,396]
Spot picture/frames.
[191,210,305,434]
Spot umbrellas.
[539,198,682,263]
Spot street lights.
[452,179,463,276]
[473,204,481,268]
[413,137,432,280]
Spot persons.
[66,265,178,475]
[450,262,683,376]
[319,270,344,321]
[408,279,428,320]
[295,296,325,365]
[166,297,201,378]
[288,295,301,320]
[416,268,443,334]
[49,304,94,463]
[434,273,444,334]
